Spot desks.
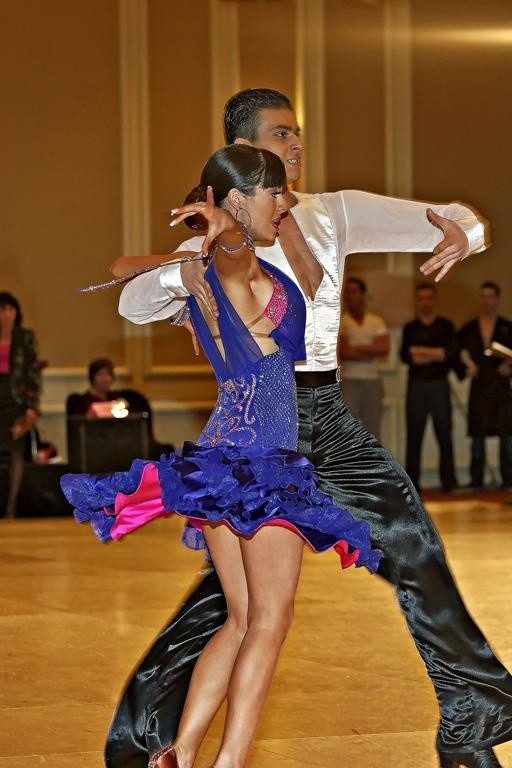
[68,411,154,473]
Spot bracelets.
[215,232,247,253]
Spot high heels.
[437,741,507,768]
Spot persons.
[398,282,458,496]
[26,423,57,465]
[66,358,151,418]
[104,88,511,767]
[54,143,382,767]
[1,290,41,518]
[338,278,389,443]
[450,283,511,488]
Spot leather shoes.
[147,746,181,768]
